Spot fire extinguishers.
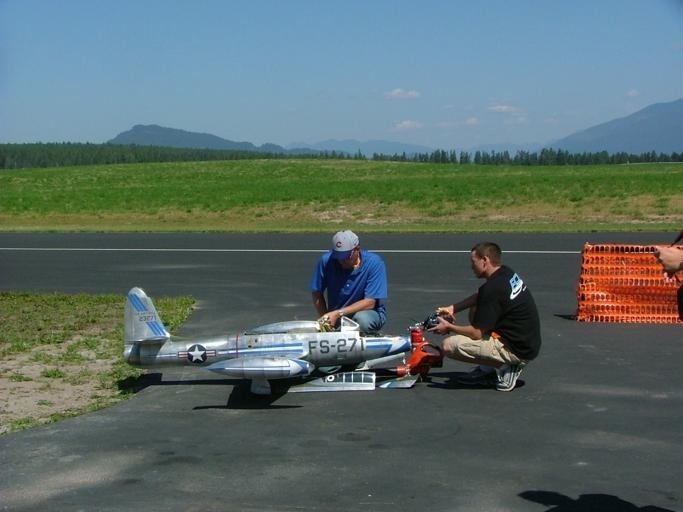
[411,322,424,374]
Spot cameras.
[426,315,452,332]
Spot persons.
[654,230,683,321]
[309,229,388,337]
[427,241,541,391]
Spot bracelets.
[339,309,344,318]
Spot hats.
[330,229,360,261]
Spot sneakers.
[495,364,524,392]
[455,366,500,387]
